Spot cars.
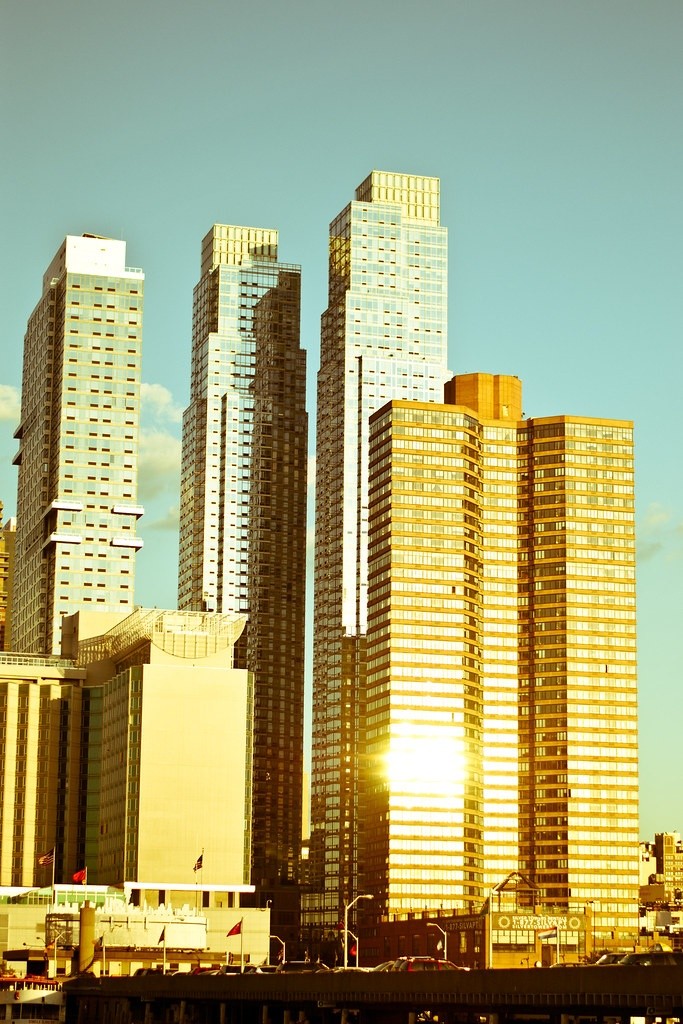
[131,953,466,978]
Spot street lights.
[488,887,499,969]
[340,929,359,967]
[343,894,374,967]
[632,897,640,946]
[102,924,121,976]
[426,923,448,961]
[589,900,596,953]
[269,935,286,965]
[54,930,72,977]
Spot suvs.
[548,951,683,968]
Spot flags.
[349,944,357,957]
[72,869,86,882]
[158,930,164,946]
[37,848,54,868]
[227,920,242,936]
[193,855,202,873]
